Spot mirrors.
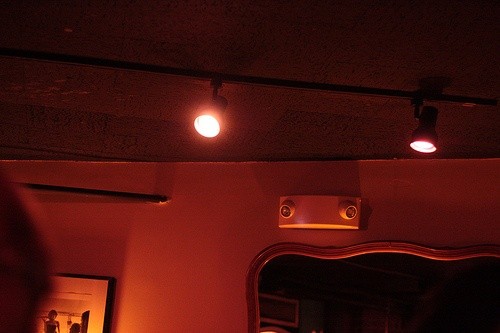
[247,240,500,333]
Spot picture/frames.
[257,291,302,330]
[33,271,118,333]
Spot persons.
[42,309,61,333]
[68,322,81,333]
[80,310,91,333]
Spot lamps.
[194,80,229,140]
[405,96,440,155]
